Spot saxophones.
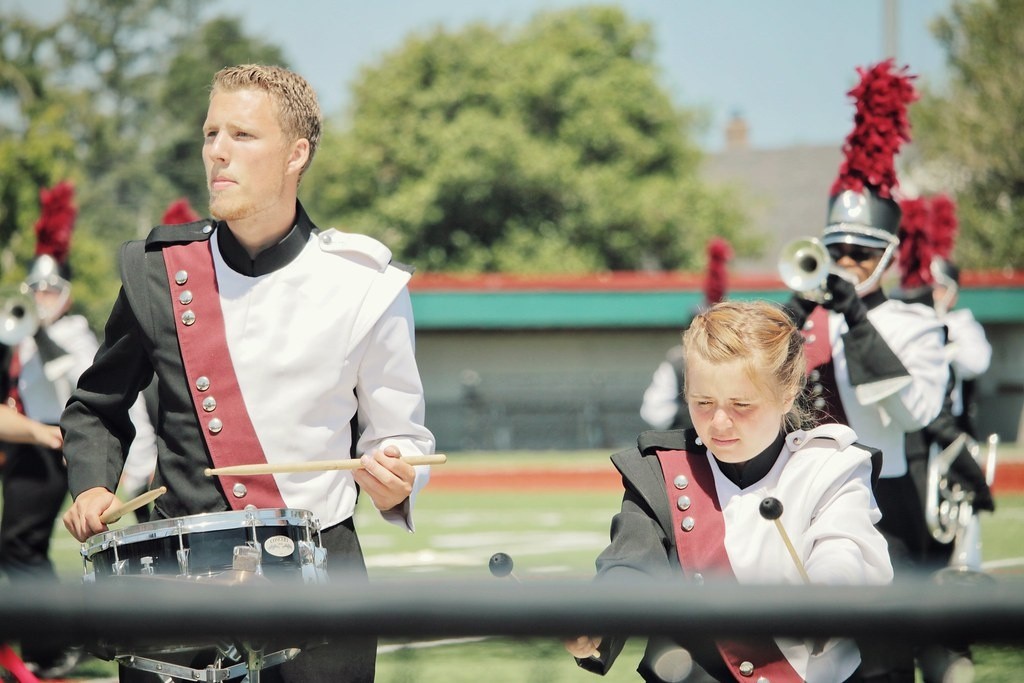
[923,260,999,545]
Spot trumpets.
[0,287,42,349]
[777,231,855,305]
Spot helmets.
[24,179,78,293]
[820,56,923,249]
[889,190,959,303]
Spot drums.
[76,504,321,661]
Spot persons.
[778,185,992,683]
[59,64,436,683]
[0,254,159,683]
[640,308,692,429]
[562,301,894,683]
